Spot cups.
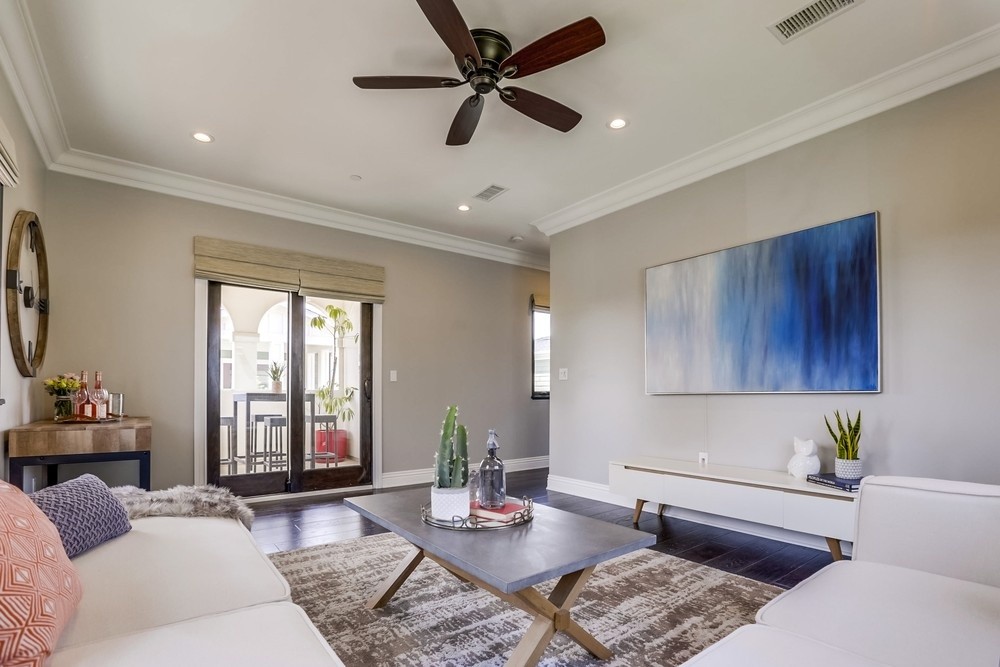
[108,393,123,419]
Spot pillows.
[28,474,133,558]
[0,478,84,667]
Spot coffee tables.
[343,484,656,667]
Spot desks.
[234,392,315,474]
[8,417,152,493]
[611,458,859,560]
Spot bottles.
[92,371,107,420]
[478,430,506,509]
[79,371,92,420]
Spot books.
[470,501,528,523]
[807,473,863,492]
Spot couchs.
[47,516,348,667]
[678,475,1000,667]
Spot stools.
[220,413,339,476]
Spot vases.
[54,396,73,420]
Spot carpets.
[266,531,791,667]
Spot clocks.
[6,210,50,377]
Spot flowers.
[42,372,80,417]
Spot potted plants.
[310,305,359,462]
[264,361,286,392]
[824,409,864,479]
[431,401,472,521]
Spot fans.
[353,0,606,146]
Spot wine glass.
[87,389,109,421]
[69,388,87,414]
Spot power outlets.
[699,452,708,464]
